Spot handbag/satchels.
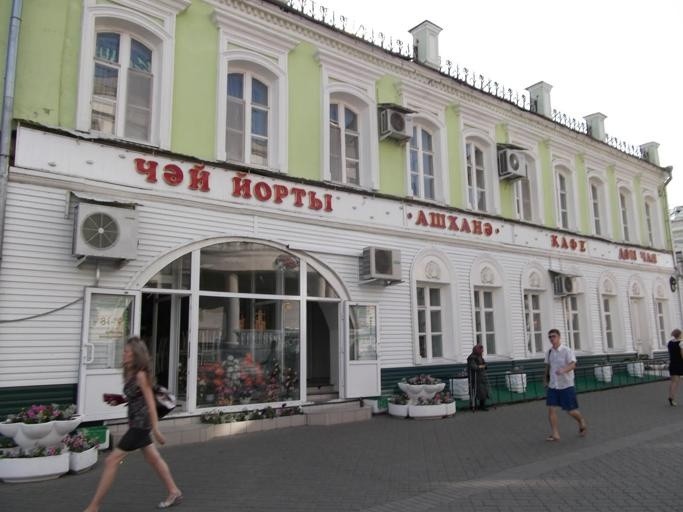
[153,384,176,418]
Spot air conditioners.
[498,149,526,182]
[360,246,402,286]
[73,200,136,266]
[553,275,577,298]
[380,108,410,144]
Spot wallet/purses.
[104,393,123,403]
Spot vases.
[203,394,252,405]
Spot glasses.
[548,335,555,338]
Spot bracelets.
[152,428,159,433]
[566,365,572,371]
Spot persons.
[467,344,490,411]
[665,329,683,407]
[81,336,181,512]
[543,329,587,441]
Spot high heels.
[158,493,183,508]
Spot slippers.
[546,436,560,441]
[580,427,586,436]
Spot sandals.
[668,398,677,406]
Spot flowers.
[1,434,96,457]
[0,403,79,425]
[388,392,453,407]
[198,353,304,393]
[396,374,445,385]
